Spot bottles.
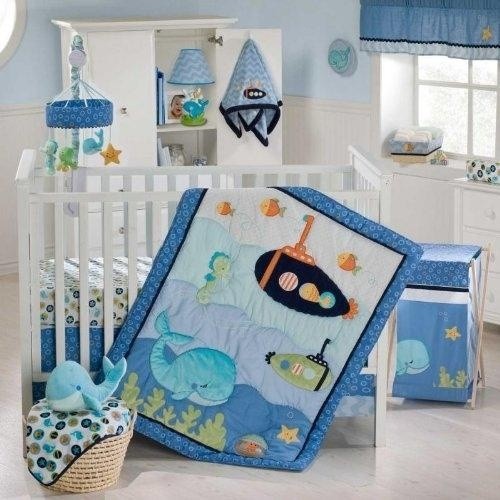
[169,143,185,166]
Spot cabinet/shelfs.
[48,14,286,260]
[458,187,499,325]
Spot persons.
[169,95,185,121]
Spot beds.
[12,141,391,449]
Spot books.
[156,137,166,167]
[164,146,173,167]
[156,67,165,128]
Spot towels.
[218,38,282,148]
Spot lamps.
[167,46,215,127]
